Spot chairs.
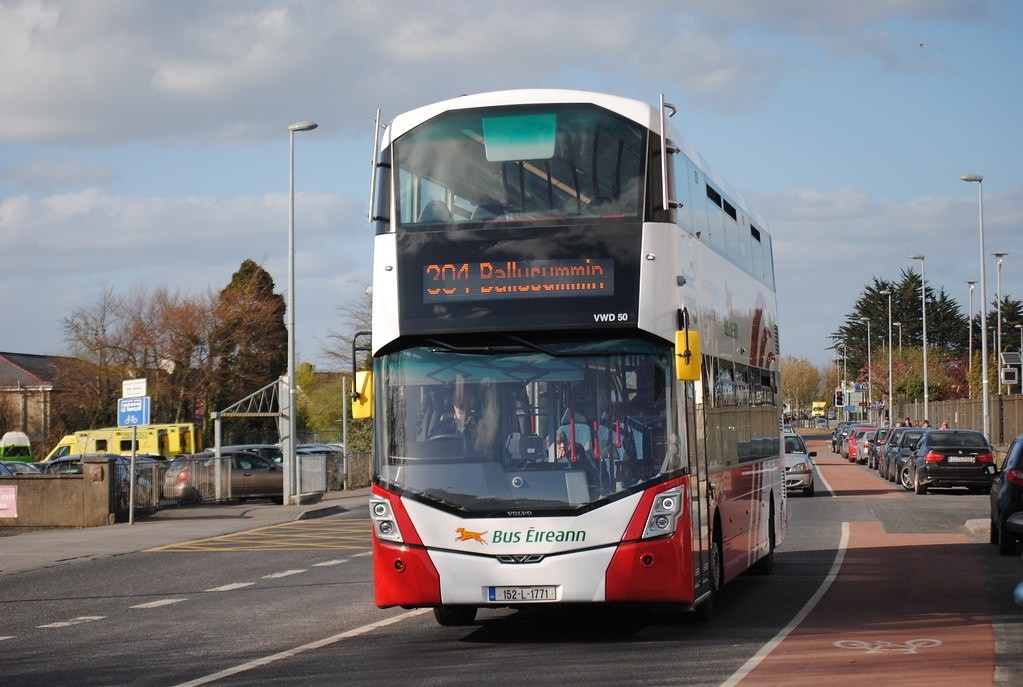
[416,200,454,222]
[471,199,506,221]
[584,196,614,216]
[439,392,477,421]
[558,406,645,479]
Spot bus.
[350,90,784,627]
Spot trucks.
[40,428,170,463]
[811,402,828,419]
[0,430,35,464]
[98,423,196,459]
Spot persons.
[914,420,920,428]
[922,420,932,429]
[896,417,912,427]
[430,386,484,450]
[883,417,889,428]
[940,422,950,430]
[597,159,638,213]
[558,431,569,457]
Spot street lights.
[893,321,902,366]
[963,280,979,399]
[838,344,847,388]
[879,290,893,427]
[910,254,929,420]
[1015,325,1023,396]
[961,173,992,447]
[278,119,320,506]
[990,251,1008,396]
[860,316,872,404]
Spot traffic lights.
[837,391,843,405]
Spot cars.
[785,433,818,499]
[981,433,1023,556]
[832,421,916,480]
[784,410,837,435]
[888,428,941,484]
[900,428,999,495]
[0,453,170,519]
[206,442,346,490]
[164,451,282,510]
